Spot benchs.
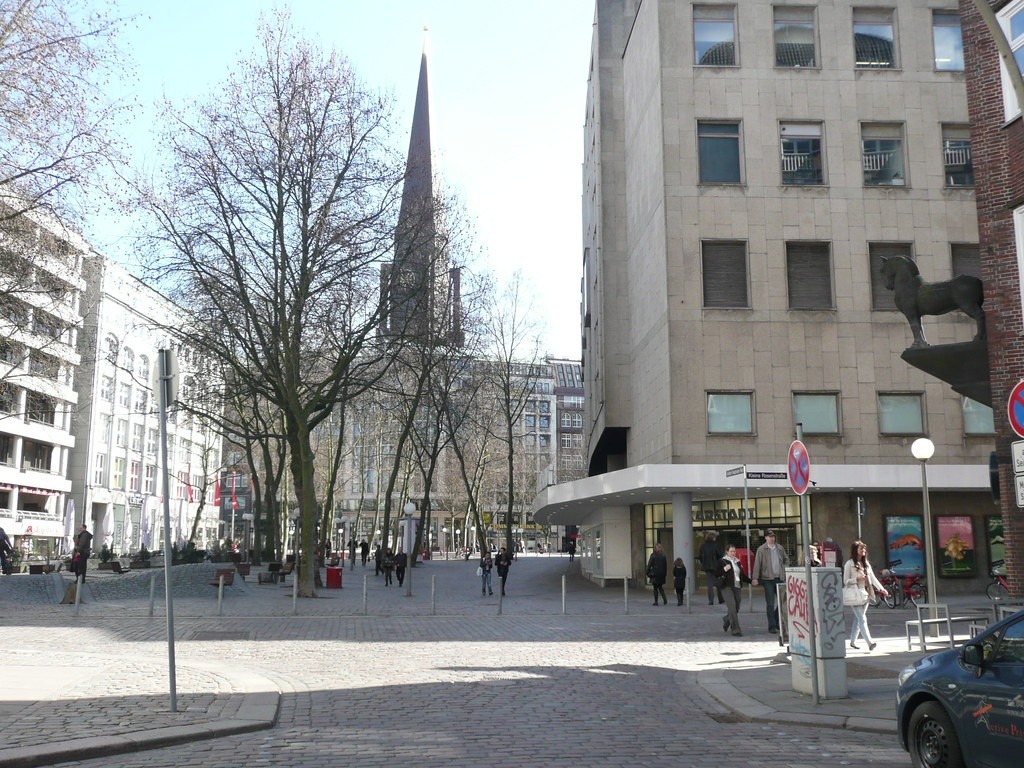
[326,557,341,566]
[906,616,989,649]
[209,562,249,598]
[464,547,473,560]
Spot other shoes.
[869,642,876,650]
[769,625,777,633]
[851,643,860,649]
[731,626,740,634]
[776,620,779,630]
[723,617,729,632]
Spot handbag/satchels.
[477,558,485,577]
[716,560,727,587]
[387,564,392,568]
[70,559,79,572]
[843,579,868,606]
[648,569,655,578]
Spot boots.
[482,588,485,595]
[653,597,658,605]
[489,587,493,595]
[662,592,667,604]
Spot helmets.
[882,569,890,577]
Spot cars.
[897,612,1024,768]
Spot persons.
[394,547,407,587]
[495,547,512,596]
[802,536,844,568]
[714,544,758,637]
[673,558,686,606]
[347,538,358,565]
[480,552,494,596]
[326,538,331,560]
[569,542,575,562]
[699,532,725,605]
[73,524,93,583]
[359,539,369,568]
[843,541,887,650]
[383,548,395,587]
[752,530,789,634]
[455,538,552,554]
[374,545,385,576]
[646,543,668,606]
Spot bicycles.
[883,566,926,609]
[866,584,896,609]
[986,564,1008,601]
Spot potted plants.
[98,543,114,569]
[5,546,24,573]
[128,547,152,568]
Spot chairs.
[259,555,297,583]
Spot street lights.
[403,502,415,597]
[242,513,254,564]
[318,515,358,571]
[292,507,301,615]
[430,526,476,560]
[910,438,941,637]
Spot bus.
[486,527,548,552]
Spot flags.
[214,474,222,506]
[188,466,194,504]
[231,473,239,510]
